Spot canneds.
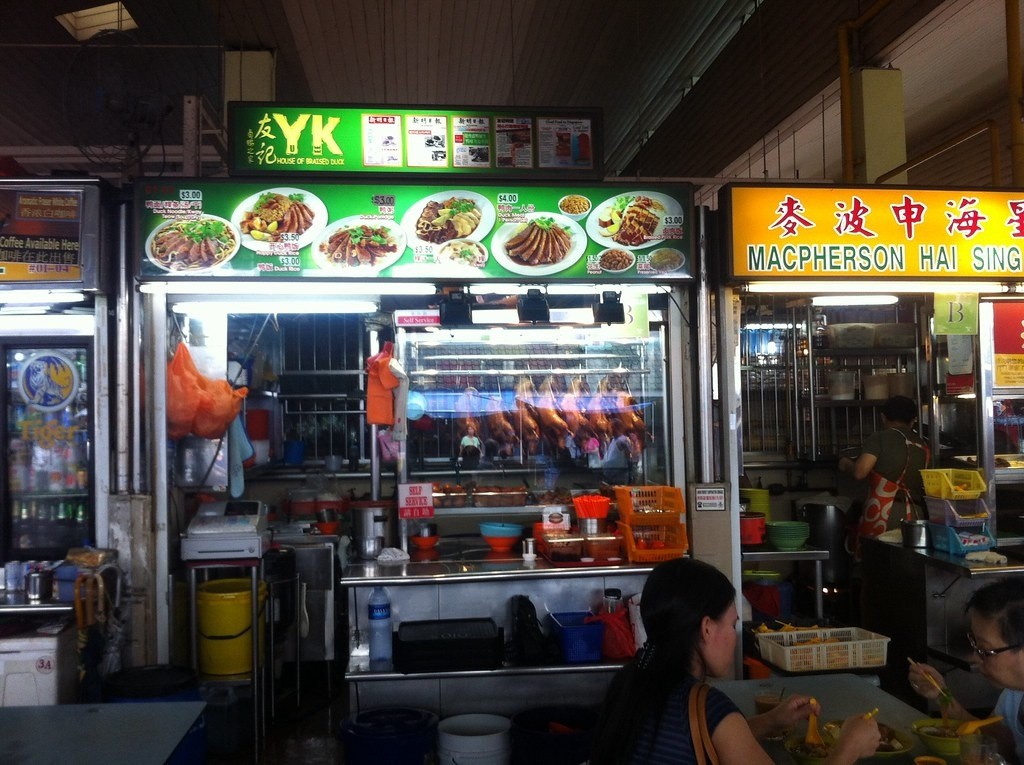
[7,352,88,492]
[4,560,53,591]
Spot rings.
[911,680,919,688]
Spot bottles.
[367,586,395,664]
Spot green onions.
[460,248,473,256]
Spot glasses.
[967,632,1019,660]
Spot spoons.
[956,716,1005,737]
[805,697,826,747]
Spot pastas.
[151,217,235,270]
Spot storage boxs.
[755,626,891,672]
[928,522,998,556]
[922,495,992,528]
[52,579,86,603]
[549,610,605,664]
[827,323,875,349]
[289,498,316,514]
[0,625,78,707]
[471,491,529,507]
[611,485,686,526]
[615,520,687,563]
[52,560,77,581]
[874,322,916,349]
[432,493,468,508]
[314,499,347,514]
[227,356,254,388]
[918,467,988,501]
[571,525,624,560]
[541,532,585,562]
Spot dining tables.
[707,673,961,765]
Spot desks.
[742,543,830,619]
[0,602,74,615]
[0,700,207,765]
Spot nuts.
[598,249,634,270]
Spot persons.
[577,427,602,469]
[458,423,486,461]
[461,445,481,471]
[601,417,639,483]
[477,434,500,470]
[835,396,932,672]
[907,574,1024,765]
[588,556,881,765]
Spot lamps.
[1010,282,1024,293]
[438,291,473,328]
[809,295,898,306]
[463,282,677,295]
[738,280,1014,294]
[515,287,552,327]
[167,301,381,315]
[135,280,444,297]
[0,292,90,303]
[590,288,626,329]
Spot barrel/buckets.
[283,440,304,465]
[829,369,917,402]
[339,703,603,765]
[325,455,343,472]
[195,577,264,674]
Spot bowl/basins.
[913,756,948,765]
[739,487,810,552]
[911,718,982,755]
[479,522,524,553]
[901,519,930,548]
[867,726,915,762]
[596,247,636,274]
[783,733,840,765]
[557,195,592,221]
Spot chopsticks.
[909,659,948,697]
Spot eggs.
[597,207,622,236]
[251,219,278,239]
[431,209,453,226]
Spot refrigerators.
[0,283,117,572]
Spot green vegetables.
[347,226,391,246]
[449,200,475,214]
[613,195,635,213]
[528,217,572,236]
[168,220,229,253]
[936,688,955,706]
[254,191,304,210]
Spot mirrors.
[741,313,801,386]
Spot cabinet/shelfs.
[273,532,340,706]
[919,293,1024,548]
[740,292,797,462]
[343,556,655,726]
[786,297,921,469]
[179,313,229,489]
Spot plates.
[433,238,488,270]
[229,187,328,253]
[490,211,588,276]
[145,212,241,273]
[401,190,496,255]
[586,190,684,251]
[311,214,407,276]
[647,248,685,273]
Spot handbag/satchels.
[583,607,635,659]
[140,343,249,440]
[629,593,648,654]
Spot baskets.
[615,485,686,563]
[548,611,602,665]
[921,469,995,557]
[757,627,891,673]
